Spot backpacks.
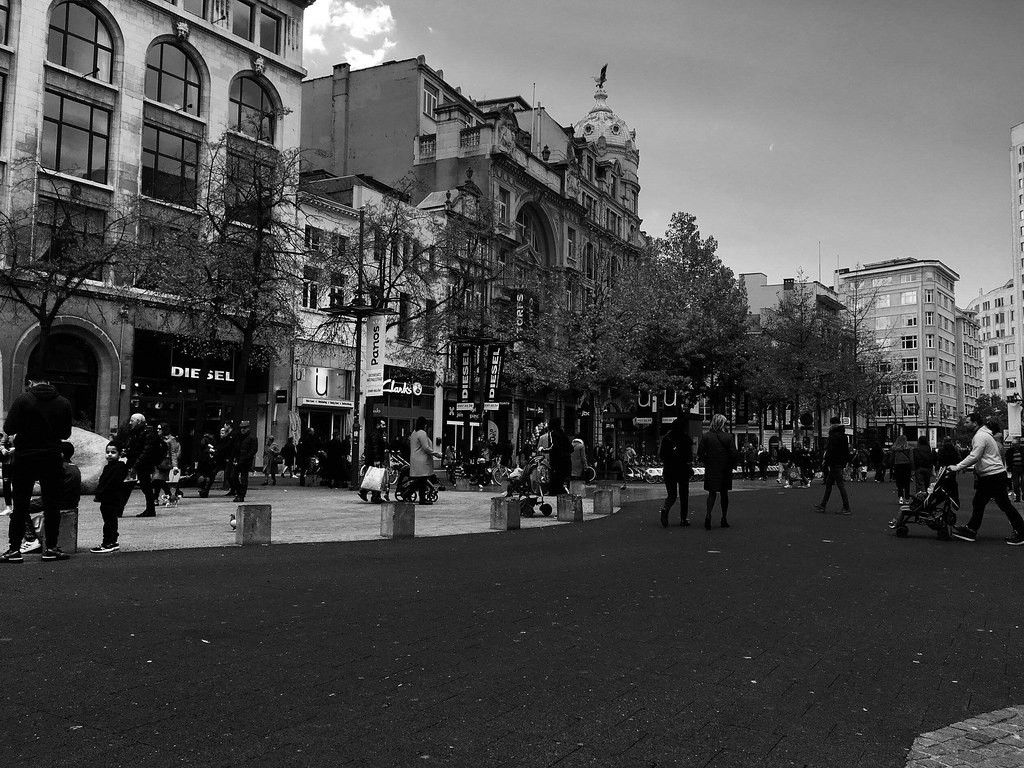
[139,429,168,467]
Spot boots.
[704,515,712,530]
[723,518,730,528]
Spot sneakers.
[952,528,976,542]
[19,538,42,553]
[1007,533,1024,545]
[41,547,70,562]
[0,549,23,563]
[90,542,120,552]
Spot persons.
[90,413,258,553]
[0,371,81,563]
[697,414,738,530]
[813,416,852,515]
[739,442,828,489]
[358,416,448,505]
[659,419,694,527]
[444,416,649,519]
[947,413,1024,545]
[260,428,351,488]
[843,435,972,511]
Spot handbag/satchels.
[360,464,387,491]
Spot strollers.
[895,464,961,541]
[389,450,445,503]
[504,450,554,519]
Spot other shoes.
[118,513,122,518]
[680,520,690,526]
[661,508,669,527]
[154,499,159,506]
[836,508,851,515]
[358,493,368,501]
[814,504,825,512]
[232,496,243,502]
[136,510,156,517]
[419,499,433,505]
[0,510,13,516]
[225,489,236,497]
[406,494,413,502]
[371,497,385,502]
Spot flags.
[873,396,947,420]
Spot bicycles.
[620,453,706,484]
[468,452,510,487]
[359,456,399,485]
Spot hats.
[240,421,250,427]
